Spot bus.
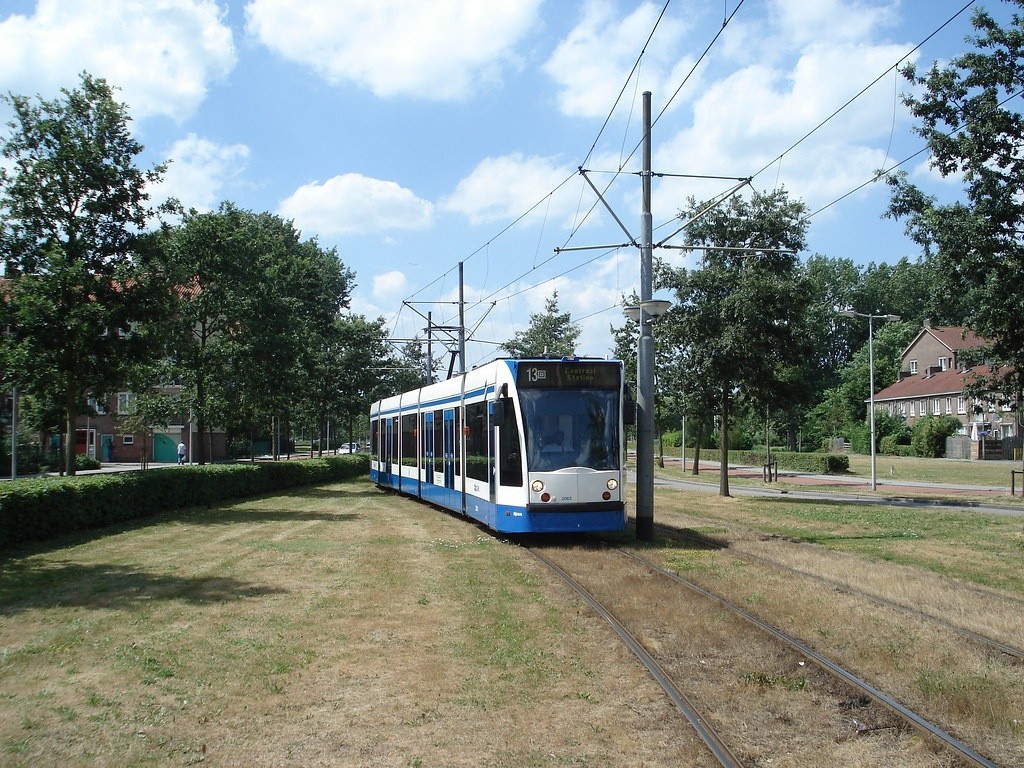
[369,356,636,534]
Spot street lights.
[839,310,901,493]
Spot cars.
[338,443,361,455]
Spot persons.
[543,431,574,451]
[106,439,113,463]
[176,441,186,465]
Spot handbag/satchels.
[181,455,187,461]
